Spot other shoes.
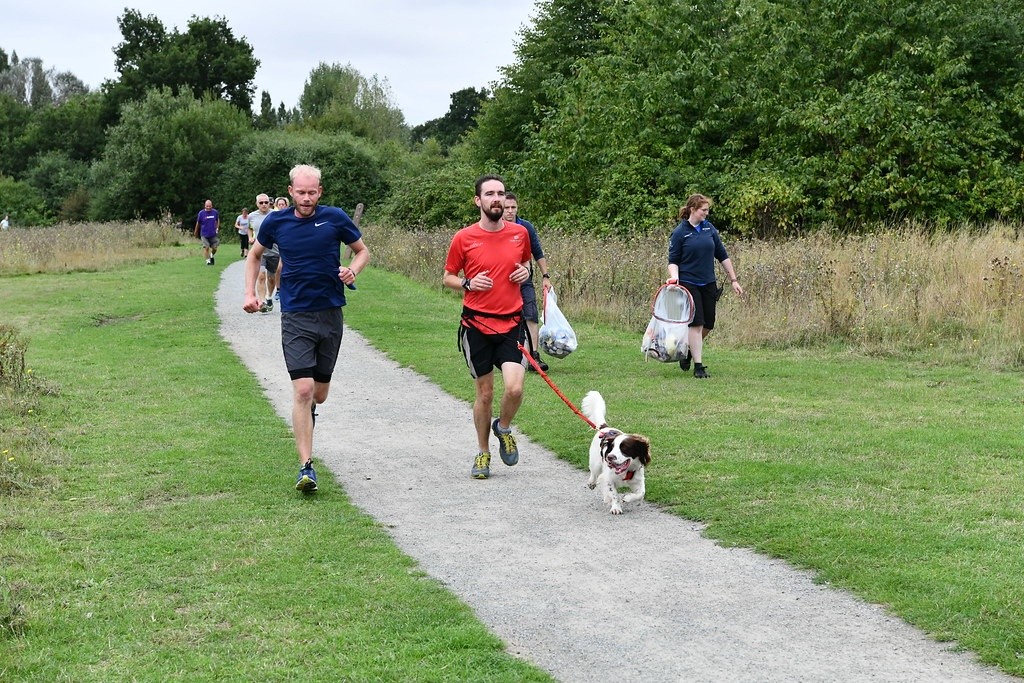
[261,303,267,312]
[244,254,247,258]
[679,346,692,372]
[693,365,710,379]
[205,259,211,265]
[274,290,280,300]
[210,252,214,258]
[267,303,273,311]
[240,250,244,257]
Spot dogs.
[580,390,651,515]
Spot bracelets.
[348,268,356,277]
[731,279,737,282]
[526,267,531,275]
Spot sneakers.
[492,419,519,466]
[295,458,318,495]
[311,401,319,429]
[528,352,548,371]
[471,452,491,478]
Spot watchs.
[461,277,472,292]
[543,273,551,278]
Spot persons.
[247,194,281,314]
[268,195,289,300]
[194,200,220,265]
[502,191,553,371]
[0,215,9,231]
[235,207,250,258]
[443,174,533,478]
[242,164,370,492]
[665,194,743,378]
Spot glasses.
[259,202,270,204]
